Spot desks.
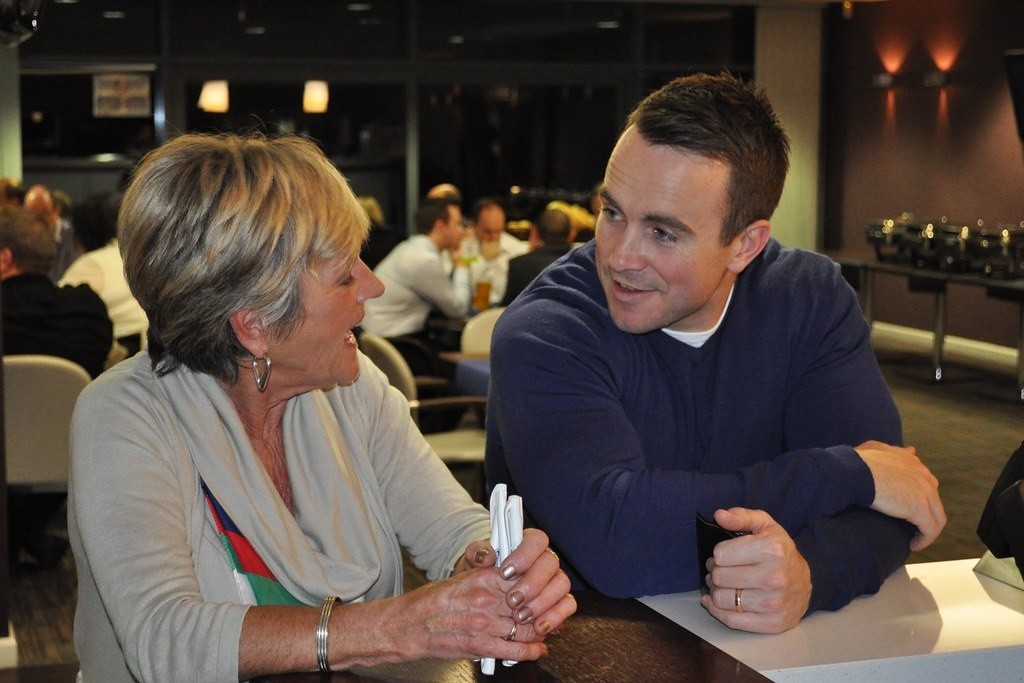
[828,247,1024,394]
[1,552,1024,683]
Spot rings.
[503,621,518,641]
[734,588,744,613]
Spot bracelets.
[317,594,343,674]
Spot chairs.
[359,331,489,505]
[1,354,96,567]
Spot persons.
[351,201,474,435]
[58,197,149,358]
[484,73,946,636]
[355,184,530,312]
[0,203,115,381]
[501,210,575,308]
[0,177,79,284]
[66,132,580,683]
